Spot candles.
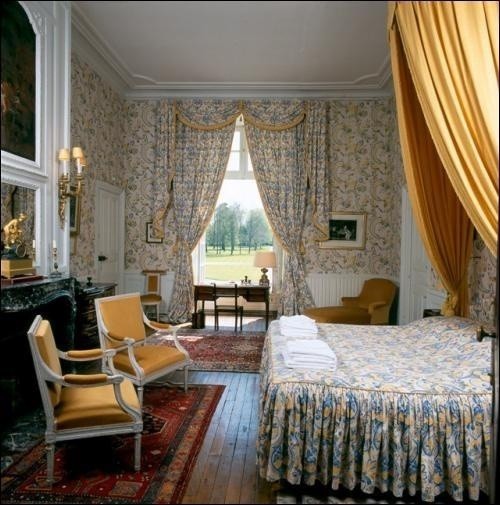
[53,240,56,248]
[32,240,36,249]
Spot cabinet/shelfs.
[74,282,117,369]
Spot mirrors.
[0,175,44,288]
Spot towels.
[279,315,318,338]
[282,339,338,373]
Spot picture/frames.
[319,211,367,250]
[145,222,163,243]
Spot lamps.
[253,251,276,286]
[57,147,87,229]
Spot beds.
[255,316,500,505]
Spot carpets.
[149,329,266,374]
[0,382,226,505]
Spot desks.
[304,306,370,324]
[193,279,271,331]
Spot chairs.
[140,270,166,323]
[94,291,193,412]
[214,284,243,331]
[27,315,143,484]
[341,278,396,325]
